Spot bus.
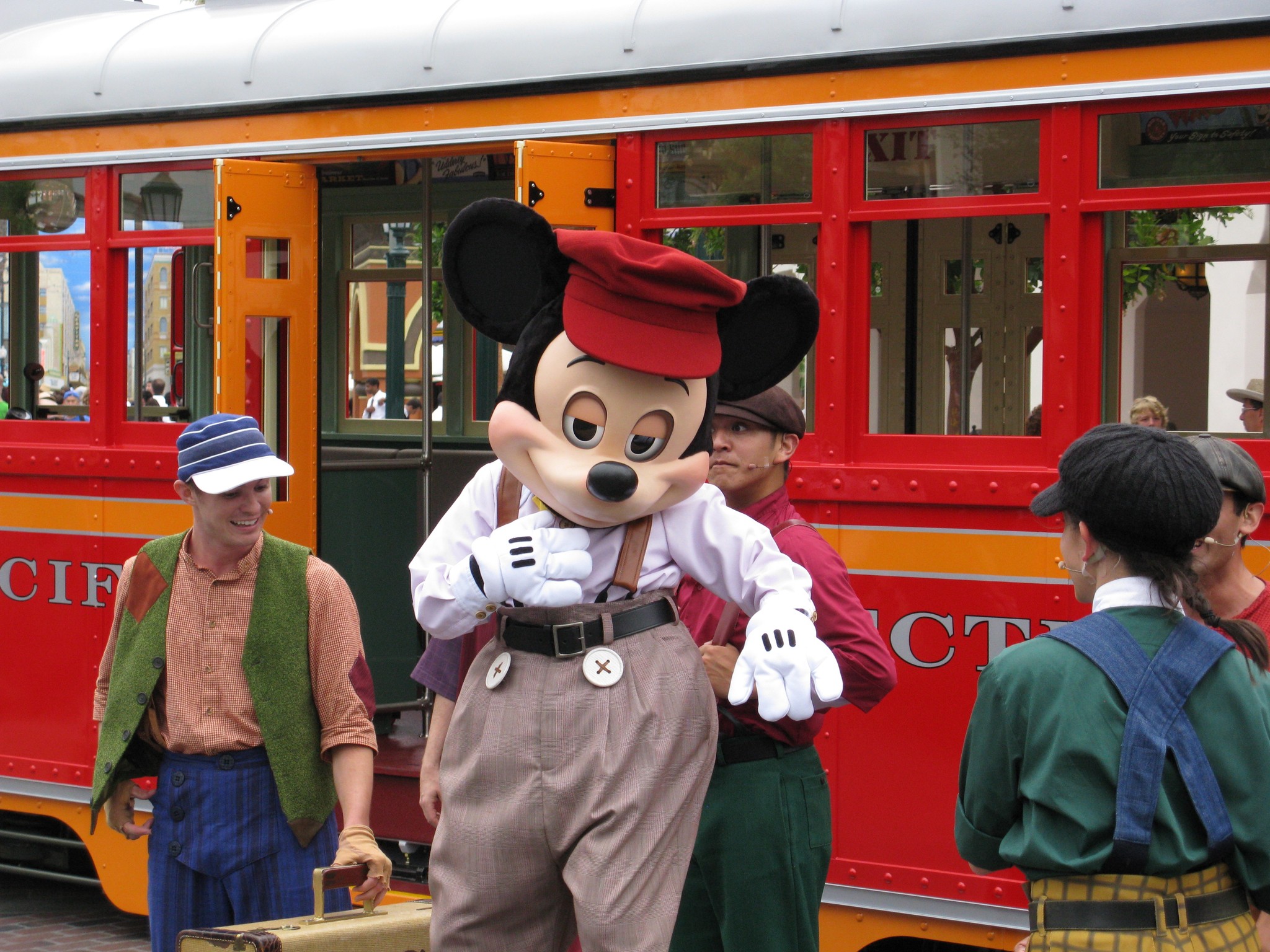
[2,1,1270,952]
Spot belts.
[1025,883,1248,933]
[715,731,815,767]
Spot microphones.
[1054,556,1082,574]
[267,508,274,515]
[747,463,776,470]
[1203,502,1253,548]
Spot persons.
[951,421,1270,952]
[1128,395,1169,430]
[0,353,445,427]
[407,193,848,952]
[1025,406,1043,436]
[1227,378,1264,430]
[1167,433,1270,675]
[671,379,901,952]
[410,634,474,827]
[88,412,393,951]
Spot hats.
[176,412,295,495]
[1226,378,1265,403]
[1181,433,1266,505]
[1028,421,1224,558]
[713,385,806,439]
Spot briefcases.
[173,863,437,952]
